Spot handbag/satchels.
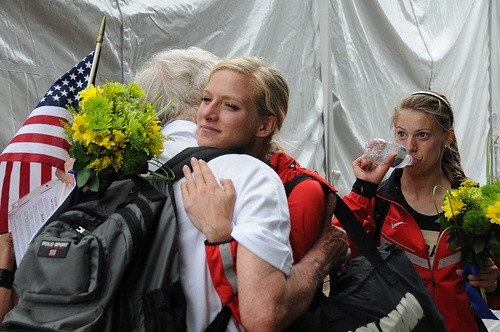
[299,244,446,332]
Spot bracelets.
[0,268,15,290]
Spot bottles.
[364,138,414,169]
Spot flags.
[0,49,97,233]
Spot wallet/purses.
[1,147,241,332]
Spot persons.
[332,88,500,332]
[57,50,329,332]
[0,234,15,324]
[66,45,347,331]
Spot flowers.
[58,80,177,193]
[433,178,500,307]
